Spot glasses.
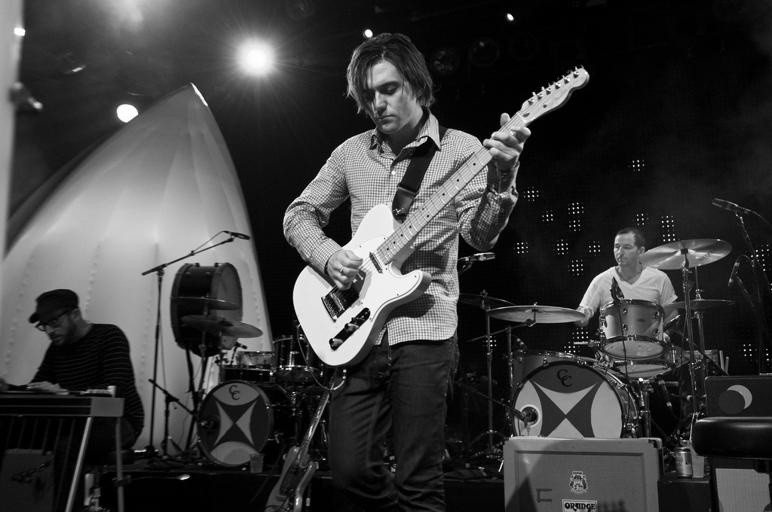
[34,310,70,332]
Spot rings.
[337,267,345,274]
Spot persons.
[23,289,144,511]
[575,229,683,362]
[280,31,534,512]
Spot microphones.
[728,255,745,287]
[612,276,624,299]
[458,252,494,262]
[711,197,751,216]
[521,406,537,420]
[224,231,250,240]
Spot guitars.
[292,65,589,368]
[265,376,338,511]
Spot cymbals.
[639,239,731,270]
[485,306,586,323]
[172,297,241,310]
[666,298,734,310]
[182,315,263,338]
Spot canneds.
[675,447,693,478]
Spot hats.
[28,290,78,323]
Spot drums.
[219,365,275,400]
[501,349,575,387]
[196,381,301,468]
[598,299,665,360]
[170,263,242,355]
[514,360,649,439]
[272,334,329,381]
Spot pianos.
[0,392,123,417]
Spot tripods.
[132,237,232,466]
[464,307,507,461]
